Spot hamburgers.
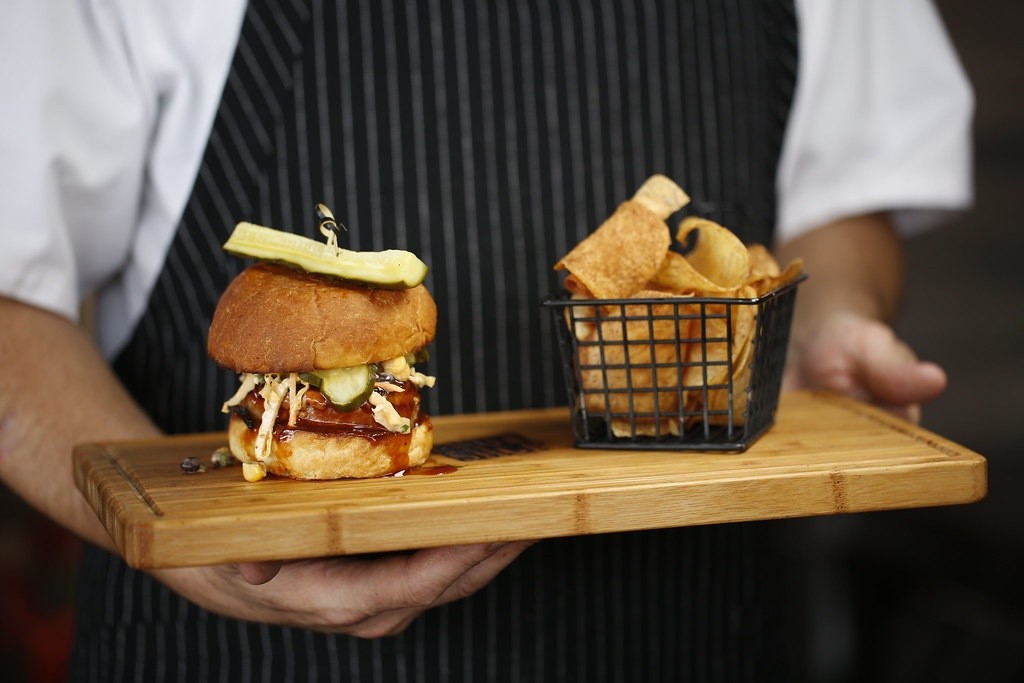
[206,220,436,481]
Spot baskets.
[541,269,814,454]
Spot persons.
[1,0,979,683]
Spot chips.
[554,172,801,436]
